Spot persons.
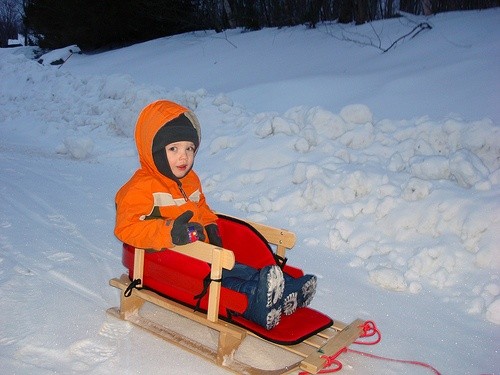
[113,99,318,331]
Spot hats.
[152,113,199,152]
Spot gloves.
[170,210,205,246]
[205,224,222,247]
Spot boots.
[222,264,285,330]
[251,268,317,316]
